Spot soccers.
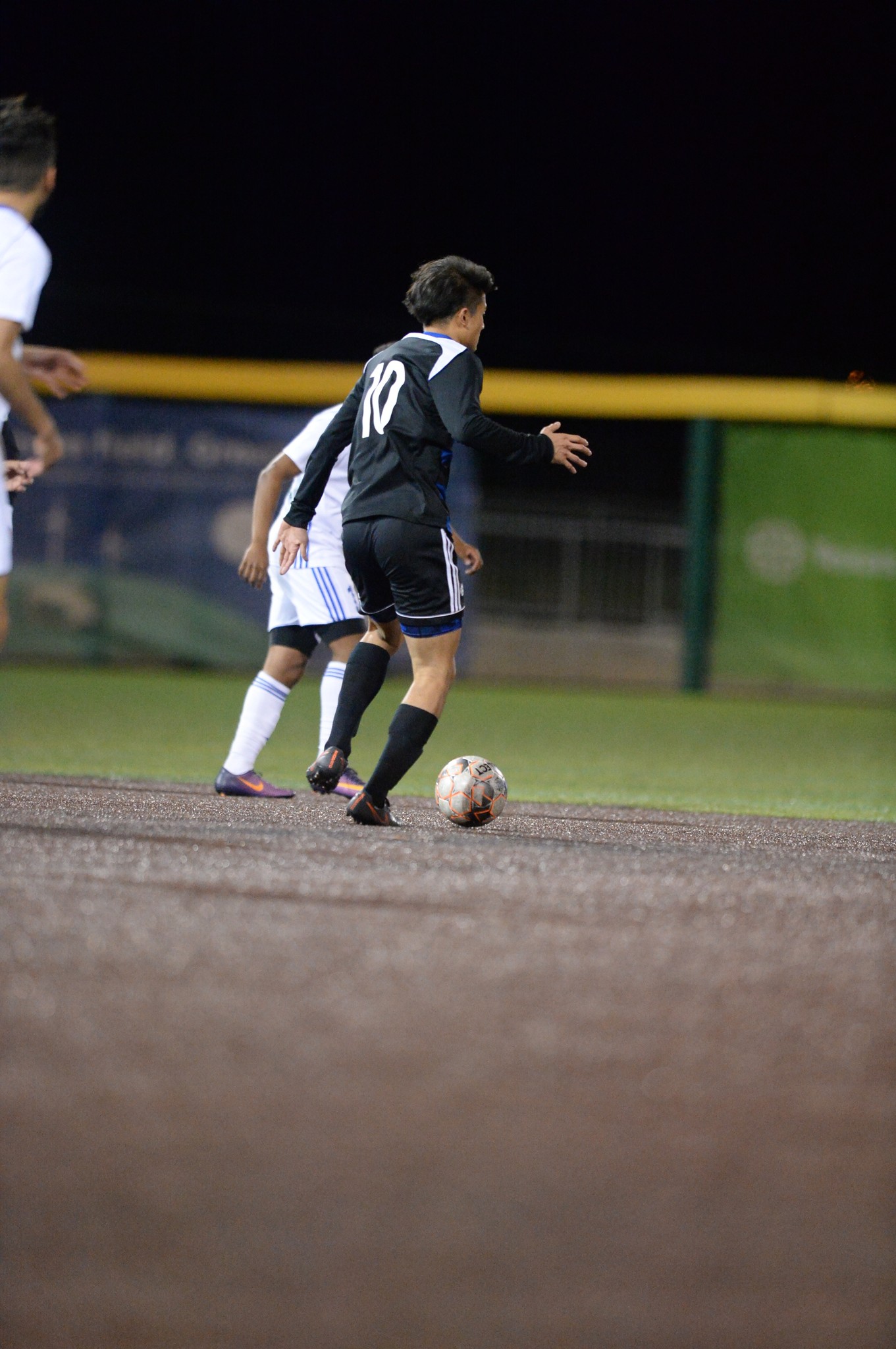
[434,755,507,826]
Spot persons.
[270,251,597,832]
[3,403,47,505]
[217,333,482,805]
[1,91,91,650]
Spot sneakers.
[346,790,402,827]
[332,767,367,798]
[306,746,348,795]
[214,766,296,798]
